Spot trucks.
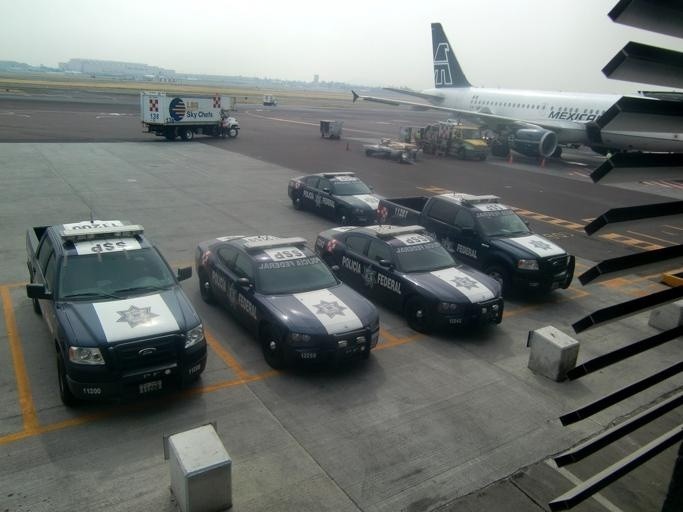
[140,90,240,142]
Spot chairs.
[66,253,158,288]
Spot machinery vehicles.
[421,123,489,161]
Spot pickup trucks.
[24,212,206,406]
[373,192,574,297]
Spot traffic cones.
[538,159,546,170]
[507,155,513,165]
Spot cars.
[194,234,379,370]
[287,171,388,226]
[314,224,504,333]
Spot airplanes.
[351,23,682,158]
